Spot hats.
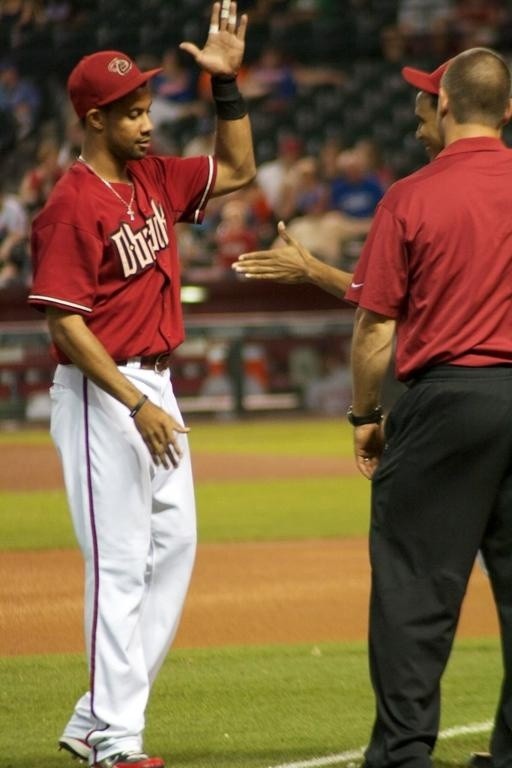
[66,49,164,122]
[401,54,453,98]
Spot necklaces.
[76,153,141,221]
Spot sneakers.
[90,749,165,768]
[58,733,92,761]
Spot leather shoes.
[468,750,492,768]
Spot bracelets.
[345,400,386,427]
[125,395,151,420]
[211,69,251,126]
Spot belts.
[109,352,175,375]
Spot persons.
[28,2,262,768]
[229,51,456,328]
[341,48,512,767]
